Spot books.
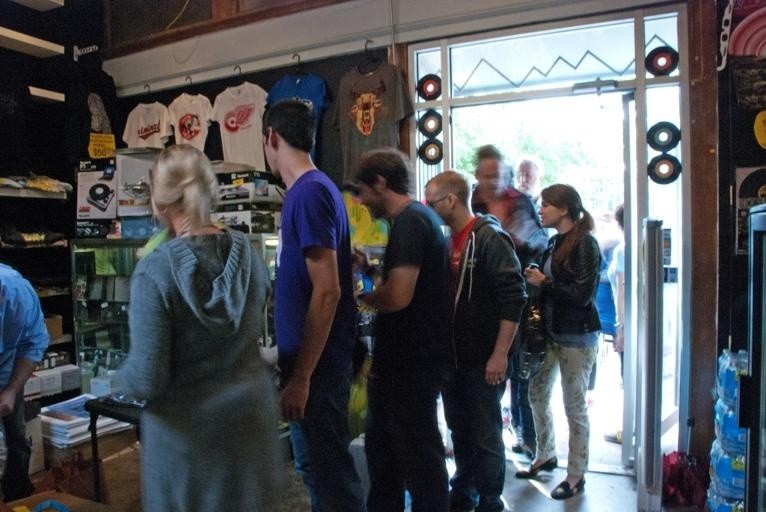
[36,392,133,448]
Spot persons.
[469,145,552,461]
[348,145,460,512]
[0,261,53,503]
[421,170,531,511]
[259,101,370,512]
[110,144,291,511]
[513,182,604,498]
[604,203,626,442]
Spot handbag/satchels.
[510,301,547,383]
[660,452,710,508]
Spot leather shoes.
[551,478,584,499]
[512,437,557,479]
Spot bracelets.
[364,264,377,277]
[354,289,369,309]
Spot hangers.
[141,84,155,102]
[228,66,245,88]
[185,76,199,96]
[288,54,311,76]
[358,40,379,70]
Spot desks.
[83,392,147,503]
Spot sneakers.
[603,431,624,444]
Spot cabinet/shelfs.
[0,68,82,408]
[0,0,65,58]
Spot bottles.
[707,348,748,512]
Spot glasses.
[427,196,448,209]
